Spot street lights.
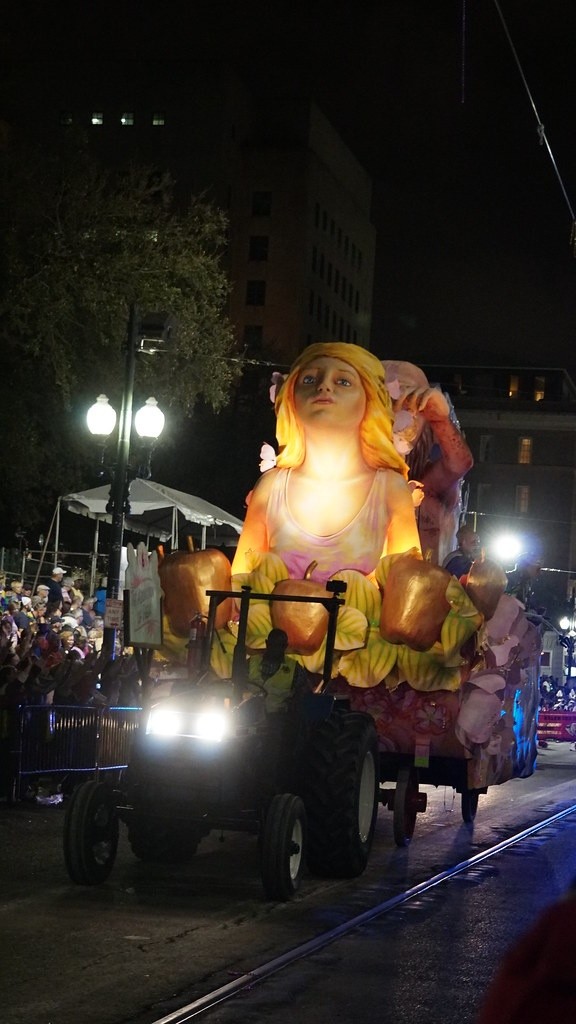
[84,390,165,707]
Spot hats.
[20,597,31,610]
[52,568,67,574]
[23,585,33,590]
[74,577,84,581]
[50,615,65,624]
[8,596,19,603]
[37,585,50,591]
[456,525,475,545]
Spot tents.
[29,475,245,604]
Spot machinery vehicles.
[63,586,488,900]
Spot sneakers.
[570,743,575,751]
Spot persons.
[1,566,154,759]
[536,673,574,753]
[504,552,543,605]
[235,627,309,738]
[230,342,475,605]
[443,525,481,579]
